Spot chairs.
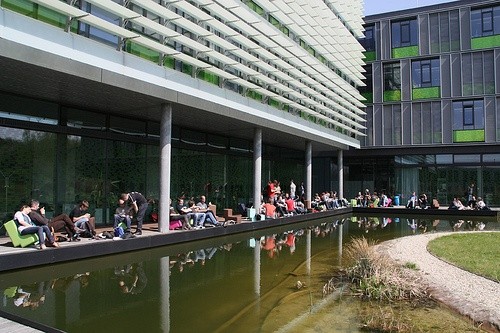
[224,209,242,224]
[209,205,225,223]
[152,214,182,230]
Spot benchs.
[4,220,46,248]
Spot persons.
[14,205,61,250]
[169,195,222,230]
[405,191,439,209]
[448,196,491,210]
[169,243,232,275]
[69,200,100,240]
[313,219,348,238]
[357,217,392,234]
[28,199,87,242]
[114,192,148,239]
[12,278,59,311]
[406,219,440,234]
[87,182,101,208]
[81,272,97,287]
[355,188,393,208]
[114,261,147,296]
[449,220,486,232]
[260,228,306,259]
[311,191,349,211]
[261,178,307,220]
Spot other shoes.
[76,229,86,234]
[40,244,46,250]
[188,225,192,230]
[51,242,59,247]
[182,226,188,230]
[134,231,142,235]
[199,224,205,228]
[216,223,223,226]
[69,237,81,242]
